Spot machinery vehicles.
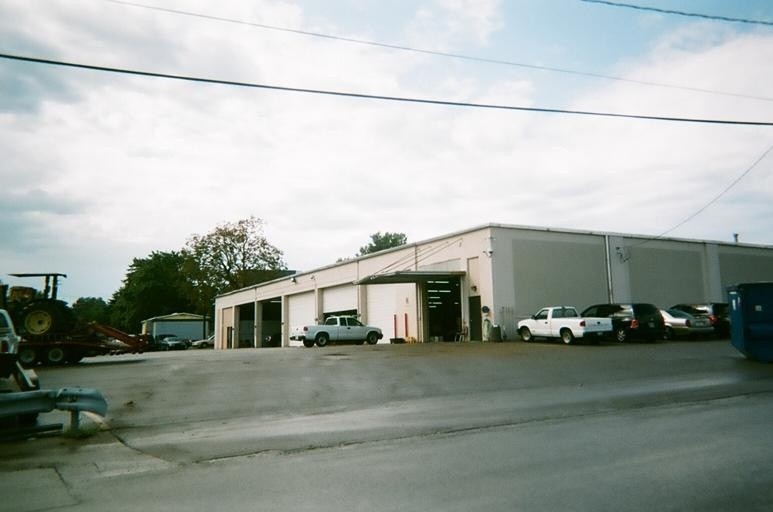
[0,273,147,367]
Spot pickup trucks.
[289,315,383,347]
[514,304,613,346]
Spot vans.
[668,303,729,337]
[580,303,665,343]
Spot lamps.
[290,278,296,284]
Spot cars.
[658,309,714,341]
[263,332,281,347]
[191,333,214,348]
[156,336,186,351]
[0,309,19,361]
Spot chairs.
[455,327,467,342]
